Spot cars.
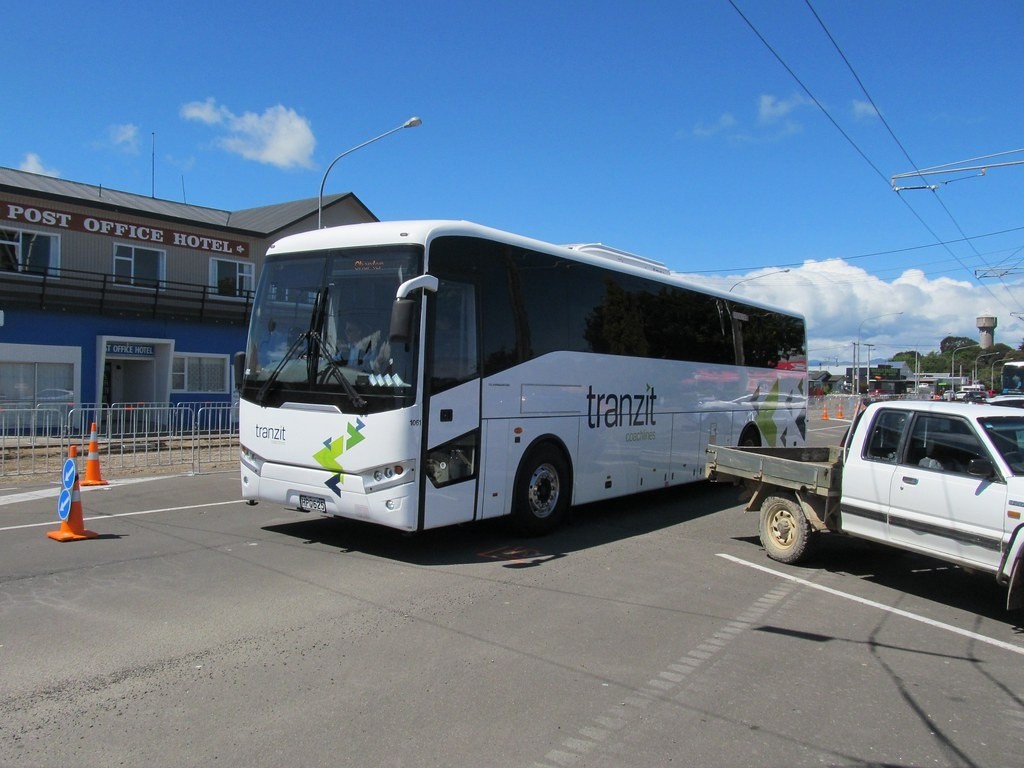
[927,360,1024,407]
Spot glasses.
[344,327,356,333]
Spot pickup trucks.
[698,407,1023,624]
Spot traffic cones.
[836,399,843,419]
[77,423,112,486]
[821,401,829,420]
[47,444,97,540]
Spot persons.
[304,319,381,374]
[930,389,945,400]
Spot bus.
[228,218,816,543]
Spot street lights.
[856,310,906,391]
[949,341,1015,398]
[729,265,790,295]
[316,112,422,229]
